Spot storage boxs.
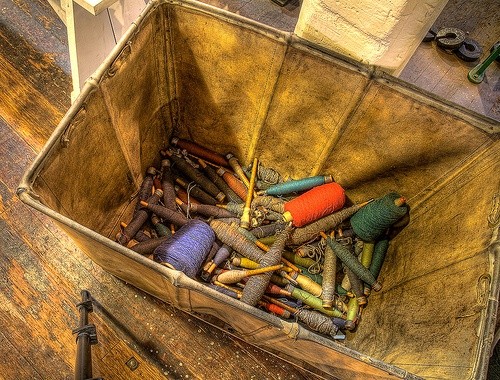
[13,0,499,380]
